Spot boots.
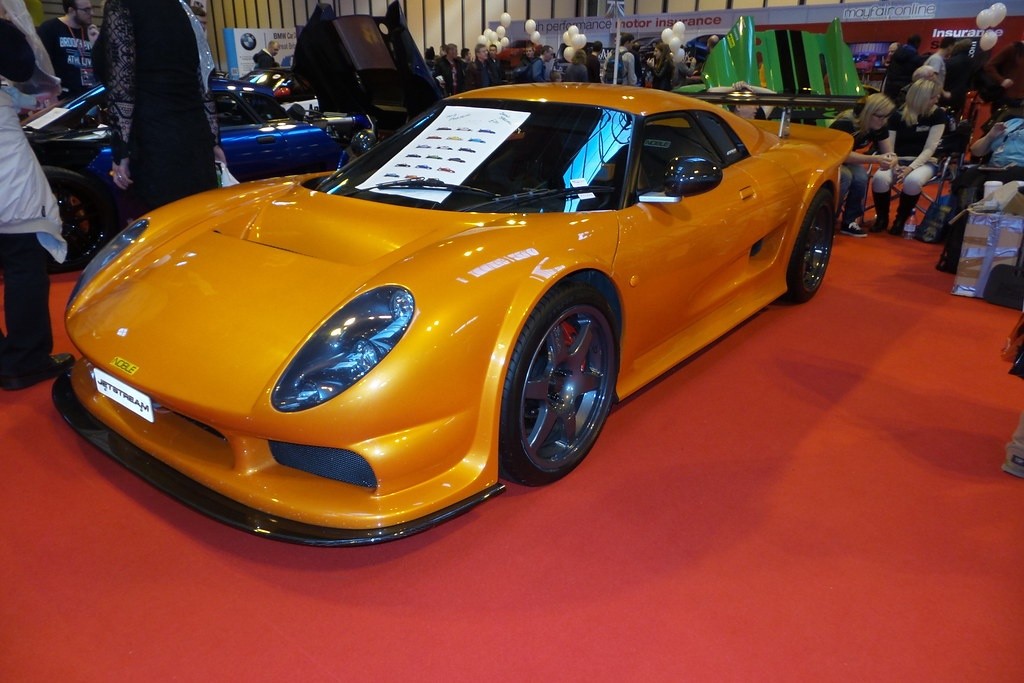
[889,188,922,235]
[870,187,892,232]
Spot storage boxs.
[949,179,1024,298]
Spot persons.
[90,0,227,237]
[423,33,718,96]
[35,0,100,100]
[1,80,77,393]
[254,39,280,68]
[825,34,1024,237]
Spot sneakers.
[1001,440,1024,479]
[841,219,868,237]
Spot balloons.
[989,2,1007,27]
[531,31,540,43]
[976,8,992,29]
[979,31,997,51]
[662,22,685,64]
[477,12,511,53]
[525,19,536,34]
[562,25,586,62]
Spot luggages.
[984,232,1024,310]
[914,157,960,244]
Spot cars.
[21,79,377,275]
[238,67,320,119]
[51,82,857,548]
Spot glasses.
[77,7,93,12]
[871,113,892,121]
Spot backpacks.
[605,49,633,83]
[518,58,543,83]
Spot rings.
[116,173,122,180]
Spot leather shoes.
[2,353,76,389]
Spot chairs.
[833,117,985,229]
[497,122,713,185]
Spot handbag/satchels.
[978,68,1006,102]
[1001,312,1024,380]
[214,162,240,189]
